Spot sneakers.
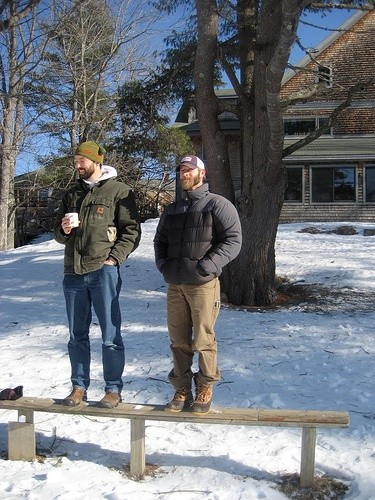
[164,386,193,412]
[63,385,87,406]
[190,386,214,416]
[97,392,122,408]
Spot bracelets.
[108,258,117,266]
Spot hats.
[175,155,205,172]
[75,141,106,166]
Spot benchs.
[0,397,349,489]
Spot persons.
[152,156,241,414]
[53,140,142,409]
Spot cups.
[64,212,79,228]
